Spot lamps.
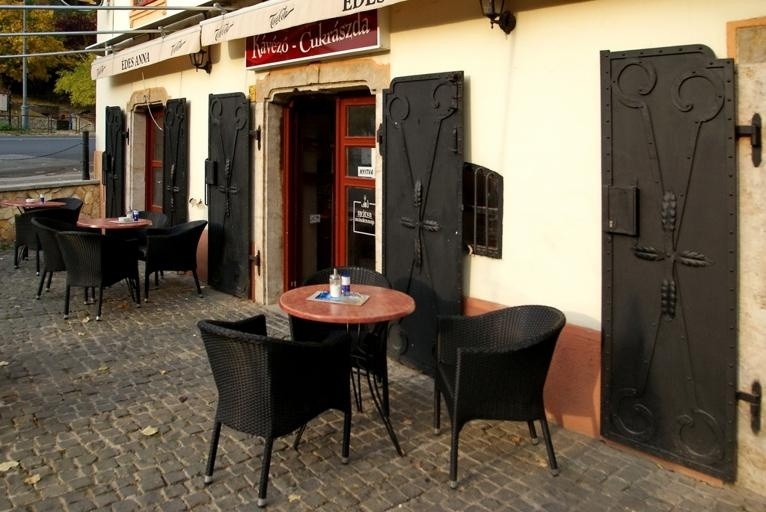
[479,0,517,34]
[189,49,211,74]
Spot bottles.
[329,273,341,297]
[127,209,139,223]
[341,270,351,296]
[40,194,44,205]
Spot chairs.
[433,303,567,490]
[198,313,354,507]
[4,194,209,320]
[304,267,395,422]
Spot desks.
[279,284,415,460]
